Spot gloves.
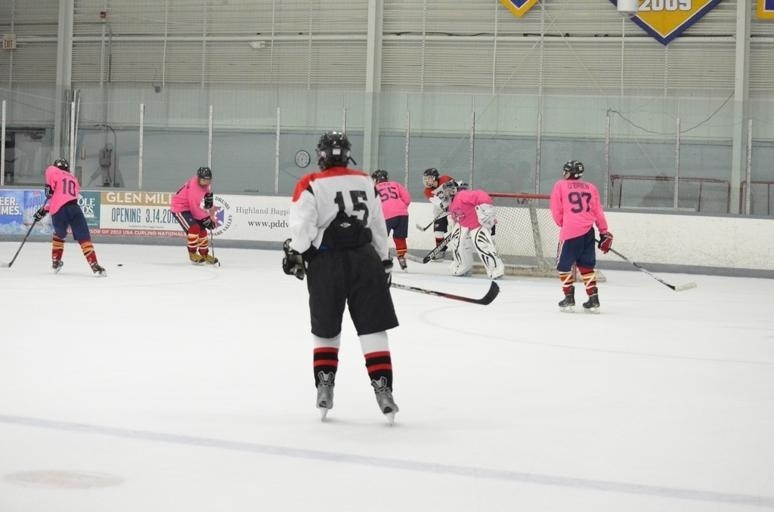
[33,206,48,222]
[282,239,305,281]
[44,183,55,200]
[382,256,394,289]
[199,215,215,230]
[598,232,615,255]
[204,192,213,209]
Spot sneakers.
[189,251,206,264]
[201,253,219,265]
[431,245,448,261]
[370,376,399,414]
[316,370,336,409]
[52,257,64,270]
[89,261,105,273]
[397,256,408,270]
[558,285,576,308]
[582,287,601,310]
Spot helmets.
[562,159,585,179]
[196,166,212,181]
[53,157,71,172]
[371,170,390,183]
[423,168,440,188]
[315,130,352,167]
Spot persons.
[278,129,402,413]
[169,166,220,265]
[440,178,504,281]
[32,159,105,273]
[369,169,412,271]
[546,159,615,307]
[420,168,465,261]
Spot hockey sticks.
[416,211,443,231]
[403,228,459,263]
[0,198,48,268]
[390,281,499,305]
[210,227,220,266]
[594,239,697,292]
